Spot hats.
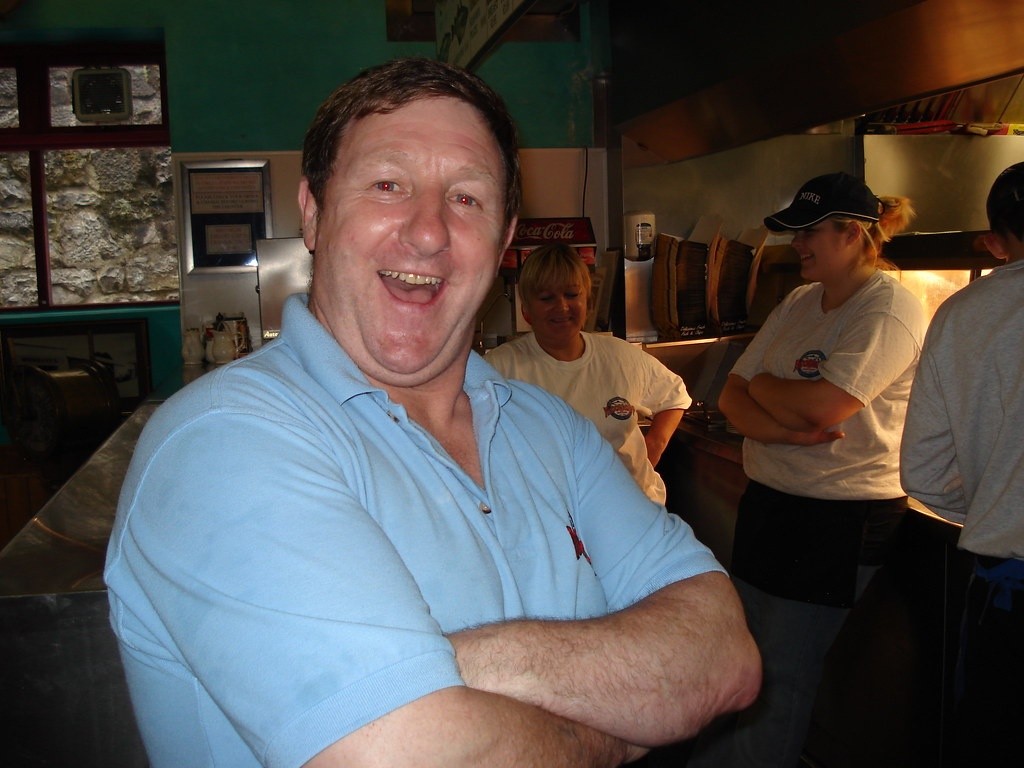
[986,162,1024,233]
[763,172,880,232]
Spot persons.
[102,53,763,768]
[719,173,1024,768]
[480,243,694,510]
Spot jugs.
[211,330,244,364]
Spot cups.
[181,332,204,365]
[206,340,216,362]
